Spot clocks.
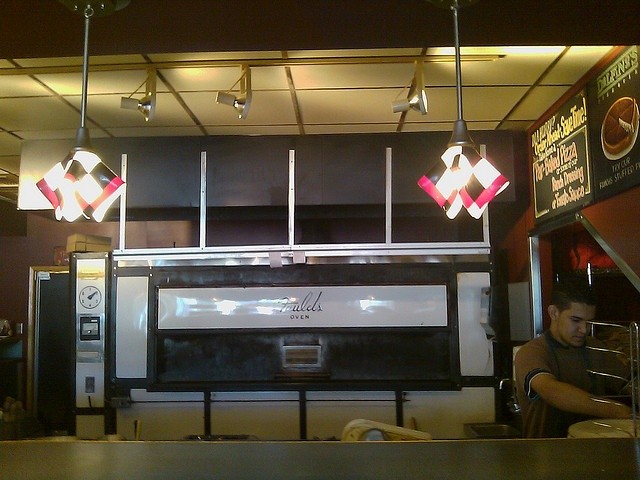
[79,286,102,309]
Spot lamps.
[119,69,157,123]
[391,61,429,116]
[35,0,126,222]
[417,0,510,220]
[215,66,253,121]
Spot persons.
[515,278,639,438]
